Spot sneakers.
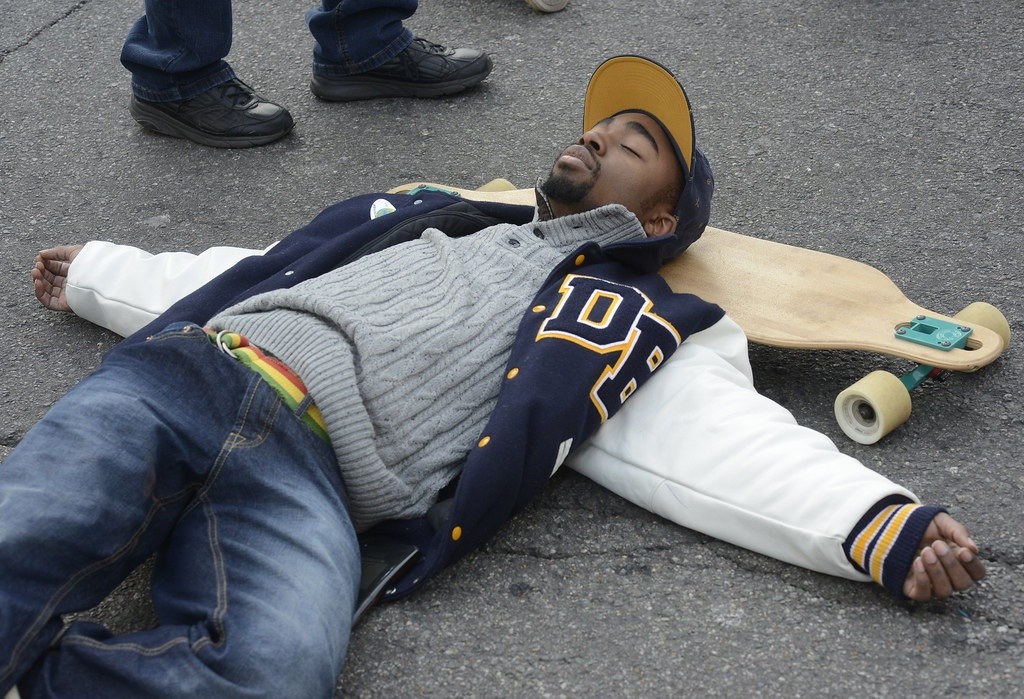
[310,35,493,101]
[128,78,297,148]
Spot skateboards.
[383,177,1013,448]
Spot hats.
[584,55,714,261]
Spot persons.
[0,55,987,699]
[121,0,492,149]
